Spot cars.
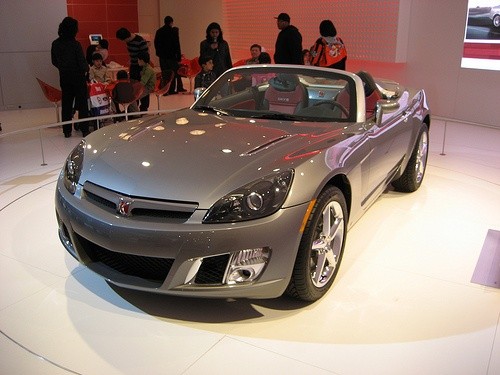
[468,0,500,33]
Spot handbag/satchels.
[87,79,110,108]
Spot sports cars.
[54,63,431,303]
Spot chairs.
[262,73,309,115]
[150,71,176,110]
[35,77,64,129]
[331,68,390,121]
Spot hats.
[273,13,290,22]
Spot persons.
[302,45,313,65]
[116,27,150,111]
[310,20,347,70]
[137,52,156,112]
[154,16,187,96]
[200,22,233,79]
[243,44,276,86]
[85,39,111,83]
[50,17,91,138]
[193,55,216,102]
[272,12,305,65]
[112,70,140,122]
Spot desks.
[107,66,128,80]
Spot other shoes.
[83,132,91,136]
[163,93,168,96]
[76,127,79,130]
[177,89,187,92]
[169,92,178,94]
[65,133,71,137]
